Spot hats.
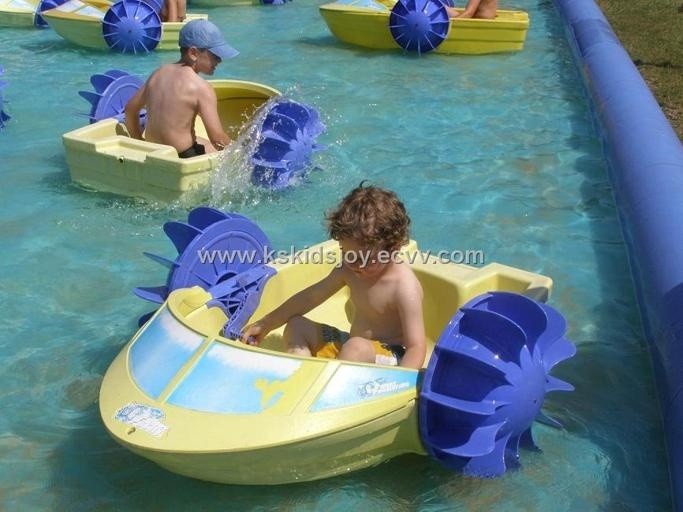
[179,19,239,59]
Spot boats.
[0,0,291,54]
[56,67,331,208]
[97,196,581,488]
[317,0,531,55]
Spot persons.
[443,1,502,23]
[238,181,428,371]
[124,16,238,158]
[156,0,189,24]
